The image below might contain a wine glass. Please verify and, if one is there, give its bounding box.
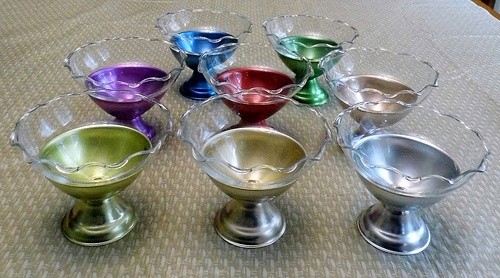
[174,93,333,248]
[10,88,171,246]
[153,8,252,100]
[334,102,490,257]
[317,47,440,146]
[261,14,361,105]
[61,37,187,138]
[197,42,312,133]
